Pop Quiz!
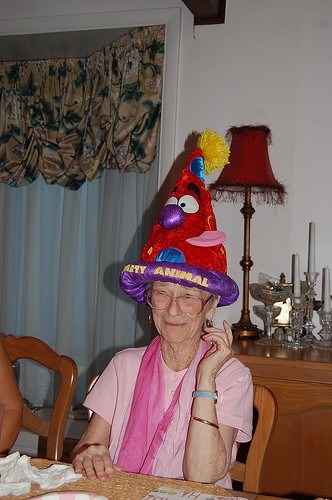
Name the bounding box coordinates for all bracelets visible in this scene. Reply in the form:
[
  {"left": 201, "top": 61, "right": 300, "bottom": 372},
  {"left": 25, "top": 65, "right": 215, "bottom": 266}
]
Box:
[{"left": 190, "top": 415, "right": 221, "bottom": 430}]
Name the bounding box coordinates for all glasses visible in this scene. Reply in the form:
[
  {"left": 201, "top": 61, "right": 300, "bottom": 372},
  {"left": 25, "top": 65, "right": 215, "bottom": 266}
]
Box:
[{"left": 147, "top": 283, "right": 213, "bottom": 316}]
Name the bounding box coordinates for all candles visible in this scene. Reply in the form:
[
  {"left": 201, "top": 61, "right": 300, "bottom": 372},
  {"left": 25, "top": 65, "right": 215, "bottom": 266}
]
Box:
[
  {"left": 309, "top": 220, "right": 315, "bottom": 274},
  {"left": 294, "top": 251, "right": 301, "bottom": 298},
  {"left": 322, "top": 266, "right": 331, "bottom": 312}
]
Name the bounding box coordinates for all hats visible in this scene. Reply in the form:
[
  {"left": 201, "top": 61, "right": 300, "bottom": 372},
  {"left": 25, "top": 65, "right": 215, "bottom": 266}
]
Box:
[{"left": 119, "top": 129, "right": 239, "bottom": 308}]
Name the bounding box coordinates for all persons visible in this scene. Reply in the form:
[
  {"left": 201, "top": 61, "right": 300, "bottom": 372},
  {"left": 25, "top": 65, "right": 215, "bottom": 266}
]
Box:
[
  {"left": 0, "top": 335, "right": 23, "bottom": 455},
  {"left": 68, "top": 265, "right": 255, "bottom": 490}
]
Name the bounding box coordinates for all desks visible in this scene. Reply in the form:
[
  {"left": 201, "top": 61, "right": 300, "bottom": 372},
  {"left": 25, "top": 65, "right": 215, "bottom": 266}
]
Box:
[
  {"left": 231, "top": 340, "right": 332, "bottom": 498},
  {"left": 0, "top": 454, "right": 275, "bottom": 500}
]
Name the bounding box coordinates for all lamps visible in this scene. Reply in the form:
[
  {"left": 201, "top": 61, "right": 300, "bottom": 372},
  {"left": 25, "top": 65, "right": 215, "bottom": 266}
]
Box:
[{"left": 207, "top": 122, "right": 288, "bottom": 339}]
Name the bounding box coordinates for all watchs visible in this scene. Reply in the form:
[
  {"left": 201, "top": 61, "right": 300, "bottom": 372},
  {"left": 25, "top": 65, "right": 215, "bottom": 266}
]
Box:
[{"left": 190, "top": 389, "right": 218, "bottom": 404}]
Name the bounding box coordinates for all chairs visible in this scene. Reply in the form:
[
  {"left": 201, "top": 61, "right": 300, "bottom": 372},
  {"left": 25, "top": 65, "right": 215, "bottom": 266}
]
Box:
[
  {"left": 3, "top": 334, "right": 79, "bottom": 460},
  {"left": 231, "top": 384, "right": 278, "bottom": 494}
]
